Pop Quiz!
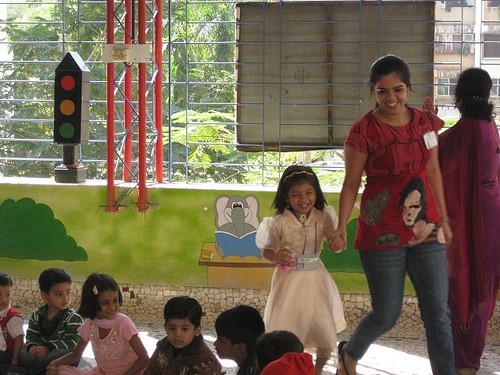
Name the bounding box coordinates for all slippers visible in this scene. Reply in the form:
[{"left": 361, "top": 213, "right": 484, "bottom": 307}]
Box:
[{"left": 335, "top": 340, "right": 356, "bottom": 375}]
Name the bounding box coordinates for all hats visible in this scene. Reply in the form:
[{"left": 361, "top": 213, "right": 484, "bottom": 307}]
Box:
[{"left": 260, "top": 353, "right": 315, "bottom": 375}]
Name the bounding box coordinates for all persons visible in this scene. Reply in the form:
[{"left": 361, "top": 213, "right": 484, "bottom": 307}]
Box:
[
  {"left": 328, "top": 53, "right": 457, "bottom": 375},
  {"left": 255, "top": 165, "right": 347, "bottom": 375},
  {"left": 213, "top": 305, "right": 265, "bottom": 375},
  {"left": 0, "top": 272, "right": 25, "bottom": 375},
  {"left": 423, "top": 68, "right": 500, "bottom": 375},
  {"left": 143, "top": 296, "right": 226, "bottom": 375},
  {"left": 18, "top": 268, "right": 85, "bottom": 375},
  {"left": 254, "top": 330, "right": 315, "bottom": 375},
  {"left": 46, "top": 273, "right": 149, "bottom": 375}
]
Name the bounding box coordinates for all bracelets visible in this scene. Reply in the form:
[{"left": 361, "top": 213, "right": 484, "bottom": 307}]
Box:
[{"left": 440, "top": 216, "right": 450, "bottom": 223}]
